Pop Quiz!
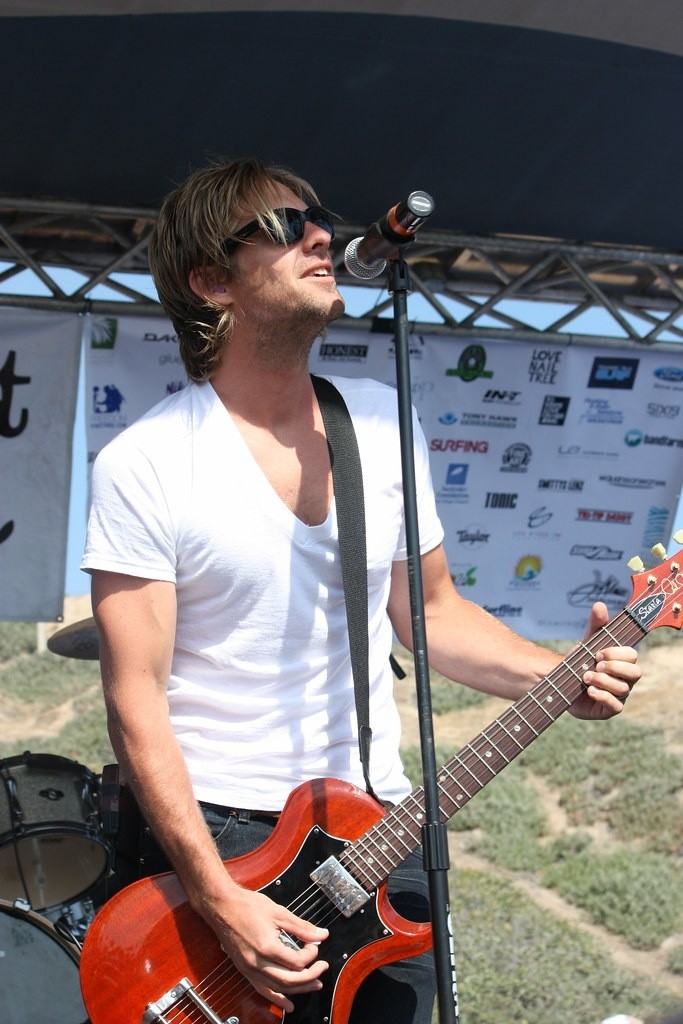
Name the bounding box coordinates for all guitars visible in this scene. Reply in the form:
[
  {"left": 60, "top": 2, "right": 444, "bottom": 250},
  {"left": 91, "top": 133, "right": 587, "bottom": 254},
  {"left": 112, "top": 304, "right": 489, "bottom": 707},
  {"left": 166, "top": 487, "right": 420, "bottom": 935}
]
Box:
[{"left": 79, "top": 527, "right": 683, "bottom": 1024}]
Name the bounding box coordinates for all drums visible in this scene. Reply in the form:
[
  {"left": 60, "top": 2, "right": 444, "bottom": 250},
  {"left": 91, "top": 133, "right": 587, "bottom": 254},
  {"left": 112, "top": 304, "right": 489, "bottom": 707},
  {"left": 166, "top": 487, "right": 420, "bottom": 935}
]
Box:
[
  {"left": 0, "top": 897, "right": 92, "bottom": 1024},
  {"left": 0, "top": 750, "right": 113, "bottom": 914}
]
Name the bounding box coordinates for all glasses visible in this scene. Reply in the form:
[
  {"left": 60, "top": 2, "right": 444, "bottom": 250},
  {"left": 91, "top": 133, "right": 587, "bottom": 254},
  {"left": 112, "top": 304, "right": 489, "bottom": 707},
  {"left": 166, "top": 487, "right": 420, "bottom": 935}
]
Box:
[{"left": 220, "top": 206, "right": 336, "bottom": 254}]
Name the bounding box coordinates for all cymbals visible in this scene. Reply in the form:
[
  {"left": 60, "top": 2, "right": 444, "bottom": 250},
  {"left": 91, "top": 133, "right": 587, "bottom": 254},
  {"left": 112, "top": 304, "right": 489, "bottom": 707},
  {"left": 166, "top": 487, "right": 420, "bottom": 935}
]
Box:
[{"left": 47, "top": 615, "right": 100, "bottom": 661}]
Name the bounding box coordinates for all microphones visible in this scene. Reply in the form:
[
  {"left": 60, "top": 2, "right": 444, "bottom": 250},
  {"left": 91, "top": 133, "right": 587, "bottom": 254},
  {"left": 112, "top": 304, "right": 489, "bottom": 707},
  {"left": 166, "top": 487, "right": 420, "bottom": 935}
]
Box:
[{"left": 344, "top": 190, "right": 435, "bottom": 279}]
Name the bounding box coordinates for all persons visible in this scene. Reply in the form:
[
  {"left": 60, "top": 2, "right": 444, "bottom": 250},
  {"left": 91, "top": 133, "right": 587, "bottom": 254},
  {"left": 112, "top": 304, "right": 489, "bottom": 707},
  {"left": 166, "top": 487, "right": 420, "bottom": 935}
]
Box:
[{"left": 80, "top": 160, "right": 642, "bottom": 1024}]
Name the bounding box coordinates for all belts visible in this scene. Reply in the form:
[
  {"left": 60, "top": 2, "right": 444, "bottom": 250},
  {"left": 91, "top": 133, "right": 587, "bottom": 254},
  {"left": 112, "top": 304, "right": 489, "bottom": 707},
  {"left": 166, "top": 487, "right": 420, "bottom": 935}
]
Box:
[{"left": 200, "top": 800, "right": 282, "bottom": 818}]
